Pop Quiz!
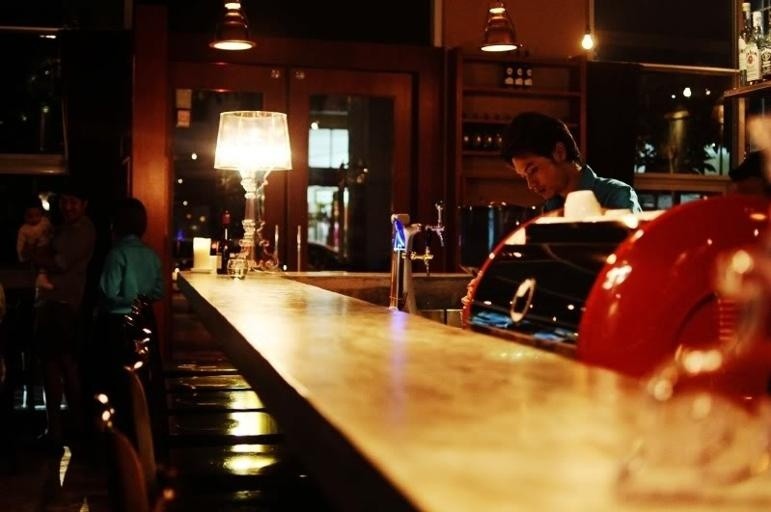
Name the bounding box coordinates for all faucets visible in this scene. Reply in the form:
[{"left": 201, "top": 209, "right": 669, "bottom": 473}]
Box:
[{"left": 390, "top": 197, "right": 448, "bottom": 312}]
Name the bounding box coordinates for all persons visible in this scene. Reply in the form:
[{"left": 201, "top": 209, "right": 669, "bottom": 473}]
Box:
[
  {"left": 501, "top": 111, "right": 643, "bottom": 213},
  {"left": 33, "top": 188, "right": 101, "bottom": 447},
  {"left": 14, "top": 201, "right": 58, "bottom": 291},
  {"left": 100, "top": 197, "right": 170, "bottom": 423}
]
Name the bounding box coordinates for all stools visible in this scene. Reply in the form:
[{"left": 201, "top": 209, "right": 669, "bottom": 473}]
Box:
[{"left": 168, "top": 347, "right": 316, "bottom": 511}]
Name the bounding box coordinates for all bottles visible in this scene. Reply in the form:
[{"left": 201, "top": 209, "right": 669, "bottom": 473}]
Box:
[
  {"left": 217, "top": 214, "right": 235, "bottom": 274},
  {"left": 738, "top": 2, "right": 754, "bottom": 88},
  {"left": 745, "top": 11, "right": 771, "bottom": 85}
]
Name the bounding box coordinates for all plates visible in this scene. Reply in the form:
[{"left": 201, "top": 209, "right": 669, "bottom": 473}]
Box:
[{"left": 191, "top": 268, "right": 213, "bottom": 273}]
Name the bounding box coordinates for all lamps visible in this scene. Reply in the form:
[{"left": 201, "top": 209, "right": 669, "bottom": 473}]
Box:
[
  {"left": 213, "top": 105, "right": 296, "bottom": 271},
  {"left": 477, "top": 0, "right": 522, "bottom": 56},
  {"left": 206, "top": 0, "right": 257, "bottom": 54},
  {"left": 579, "top": 0, "right": 596, "bottom": 52}
]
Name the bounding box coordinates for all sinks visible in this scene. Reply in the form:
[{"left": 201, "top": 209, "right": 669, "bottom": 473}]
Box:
[{"left": 278, "top": 267, "right": 477, "bottom": 315}]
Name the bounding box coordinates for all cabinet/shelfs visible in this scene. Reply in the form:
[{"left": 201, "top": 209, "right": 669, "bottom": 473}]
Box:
[
  {"left": 723, "top": 0, "right": 771, "bottom": 185},
  {"left": 451, "top": 51, "right": 591, "bottom": 272},
  {"left": 585, "top": 59, "right": 745, "bottom": 207}
]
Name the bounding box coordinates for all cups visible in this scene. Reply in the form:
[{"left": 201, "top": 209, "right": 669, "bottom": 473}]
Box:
[
  {"left": 192, "top": 237, "right": 211, "bottom": 268},
  {"left": 227, "top": 259, "right": 248, "bottom": 279}
]
